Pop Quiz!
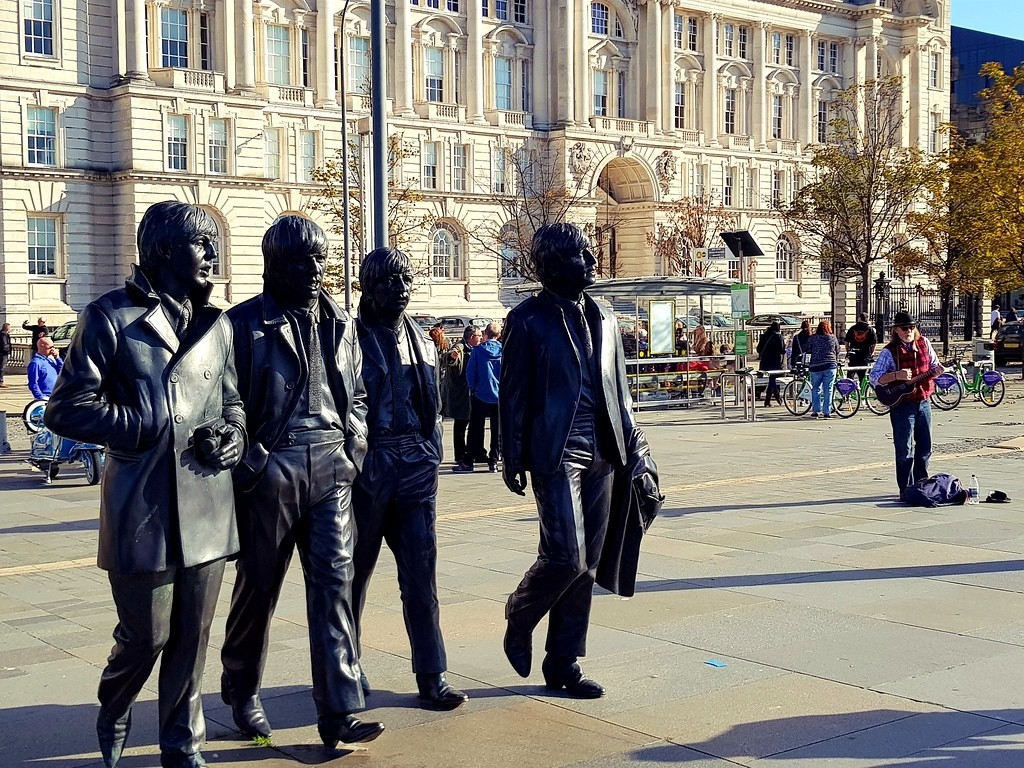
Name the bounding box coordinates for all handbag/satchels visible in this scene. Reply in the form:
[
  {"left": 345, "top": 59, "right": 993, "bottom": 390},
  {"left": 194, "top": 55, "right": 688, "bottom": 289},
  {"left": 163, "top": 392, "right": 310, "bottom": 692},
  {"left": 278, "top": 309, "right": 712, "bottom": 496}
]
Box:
[{"left": 903, "top": 473, "right": 970, "bottom": 507}]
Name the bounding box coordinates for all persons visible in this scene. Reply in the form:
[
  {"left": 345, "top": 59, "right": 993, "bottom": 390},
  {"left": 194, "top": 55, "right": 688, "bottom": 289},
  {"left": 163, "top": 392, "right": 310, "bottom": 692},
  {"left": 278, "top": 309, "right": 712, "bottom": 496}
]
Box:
[
  {"left": 756, "top": 321, "right": 786, "bottom": 408},
  {"left": 225, "top": 216, "right": 385, "bottom": 750},
  {"left": 22, "top": 316, "right": 49, "bottom": 361},
  {"left": 27, "top": 337, "right": 64, "bottom": 457},
  {"left": 42, "top": 200, "right": 249, "bottom": 768},
  {"left": 622, "top": 322, "right": 743, "bottom": 397},
  {"left": 350, "top": 247, "right": 469, "bottom": 705},
  {"left": 805, "top": 321, "right": 841, "bottom": 420},
  {"left": 844, "top": 313, "right": 877, "bottom": 387},
  {"left": 868, "top": 312, "right": 945, "bottom": 501},
  {"left": 430, "top": 322, "right": 502, "bottom": 473},
  {"left": 499, "top": 223, "right": 665, "bottom": 697},
  {"left": 990, "top": 304, "right": 1018, "bottom": 339},
  {"left": 0, "top": 323, "right": 12, "bottom": 388},
  {"left": 790, "top": 320, "right": 812, "bottom": 407}
]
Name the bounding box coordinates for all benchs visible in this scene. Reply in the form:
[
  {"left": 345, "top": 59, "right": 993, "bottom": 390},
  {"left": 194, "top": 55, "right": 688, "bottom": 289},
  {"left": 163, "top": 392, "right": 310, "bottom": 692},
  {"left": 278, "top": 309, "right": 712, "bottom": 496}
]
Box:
[{"left": 629, "top": 369, "right": 728, "bottom": 408}]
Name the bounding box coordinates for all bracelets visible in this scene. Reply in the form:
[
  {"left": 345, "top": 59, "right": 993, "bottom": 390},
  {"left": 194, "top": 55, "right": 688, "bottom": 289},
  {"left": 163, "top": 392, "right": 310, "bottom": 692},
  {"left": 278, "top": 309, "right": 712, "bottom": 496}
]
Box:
[{"left": 894, "top": 372, "right": 897, "bottom": 380}]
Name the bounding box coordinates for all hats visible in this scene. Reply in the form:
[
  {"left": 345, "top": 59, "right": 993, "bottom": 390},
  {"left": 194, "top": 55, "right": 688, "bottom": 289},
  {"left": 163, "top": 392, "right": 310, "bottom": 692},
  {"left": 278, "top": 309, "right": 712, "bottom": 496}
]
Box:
[{"left": 892, "top": 312, "right": 919, "bottom": 325}]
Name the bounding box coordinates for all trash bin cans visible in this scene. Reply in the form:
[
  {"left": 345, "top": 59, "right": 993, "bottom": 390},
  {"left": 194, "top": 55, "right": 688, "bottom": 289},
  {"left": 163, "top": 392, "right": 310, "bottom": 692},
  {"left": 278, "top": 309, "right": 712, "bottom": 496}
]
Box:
[
  {"left": 972, "top": 337, "right": 995, "bottom": 383},
  {"left": 0, "top": 411, "right": 11, "bottom": 454}
]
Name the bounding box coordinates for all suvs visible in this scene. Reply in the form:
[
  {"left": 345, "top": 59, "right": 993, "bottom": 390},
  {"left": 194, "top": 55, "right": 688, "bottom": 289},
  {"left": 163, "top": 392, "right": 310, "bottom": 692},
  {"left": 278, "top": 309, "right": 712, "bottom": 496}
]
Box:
[{"left": 411, "top": 314, "right": 498, "bottom": 346}]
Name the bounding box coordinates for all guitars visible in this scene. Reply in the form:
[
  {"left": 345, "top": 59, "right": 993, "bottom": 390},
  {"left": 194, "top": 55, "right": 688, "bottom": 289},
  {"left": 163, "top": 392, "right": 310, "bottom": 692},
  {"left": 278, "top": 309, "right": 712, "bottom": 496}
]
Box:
[{"left": 875, "top": 358, "right": 961, "bottom": 408}]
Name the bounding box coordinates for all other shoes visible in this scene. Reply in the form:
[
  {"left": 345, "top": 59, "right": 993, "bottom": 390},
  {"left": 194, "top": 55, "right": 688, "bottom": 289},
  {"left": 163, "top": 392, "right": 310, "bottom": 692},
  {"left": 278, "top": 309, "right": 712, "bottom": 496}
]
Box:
[
  {"left": 0, "top": 384, "right": 8, "bottom": 389},
  {"left": 823, "top": 414, "right": 834, "bottom": 419},
  {"left": 475, "top": 456, "right": 490, "bottom": 462},
  {"left": 811, "top": 413, "right": 818, "bottom": 419},
  {"left": 457, "top": 459, "right": 463, "bottom": 464}
]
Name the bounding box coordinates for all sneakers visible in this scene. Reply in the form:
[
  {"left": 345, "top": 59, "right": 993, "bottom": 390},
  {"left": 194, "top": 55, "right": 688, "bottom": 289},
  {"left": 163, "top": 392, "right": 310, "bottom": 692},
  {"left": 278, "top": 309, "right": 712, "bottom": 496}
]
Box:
[
  {"left": 451, "top": 463, "right": 474, "bottom": 472},
  {"left": 490, "top": 467, "right": 498, "bottom": 473}
]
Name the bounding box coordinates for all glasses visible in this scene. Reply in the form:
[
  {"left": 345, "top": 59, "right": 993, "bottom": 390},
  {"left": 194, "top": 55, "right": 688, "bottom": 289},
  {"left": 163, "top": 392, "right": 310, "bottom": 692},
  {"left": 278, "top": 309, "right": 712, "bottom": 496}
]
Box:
[
  {"left": 474, "top": 334, "right": 481, "bottom": 336},
  {"left": 897, "top": 325, "right": 915, "bottom": 331}
]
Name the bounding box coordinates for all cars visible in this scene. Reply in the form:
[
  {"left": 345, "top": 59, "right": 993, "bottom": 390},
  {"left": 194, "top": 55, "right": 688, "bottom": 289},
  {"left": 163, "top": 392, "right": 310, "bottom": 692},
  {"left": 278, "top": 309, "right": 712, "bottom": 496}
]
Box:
[
  {"left": 993, "top": 310, "right": 1024, "bottom": 367},
  {"left": 50, "top": 321, "right": 78, "bottom": 353},
  {"left": 675, "top": 314, "right": 734, "bottom": 330},
  {"left": 745, "top": 314, "right": 803, "bottom": 326}
]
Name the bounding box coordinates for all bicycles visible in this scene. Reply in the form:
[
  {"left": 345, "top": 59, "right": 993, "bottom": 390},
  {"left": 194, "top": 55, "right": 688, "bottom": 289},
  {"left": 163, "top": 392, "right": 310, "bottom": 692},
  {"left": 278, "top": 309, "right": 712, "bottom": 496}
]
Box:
[
  {"left": 783, "top": 361, "right": 861, "bottom": 418},
  {"left": 861, "top": 344, "right": 1006, "bottom": 416}
]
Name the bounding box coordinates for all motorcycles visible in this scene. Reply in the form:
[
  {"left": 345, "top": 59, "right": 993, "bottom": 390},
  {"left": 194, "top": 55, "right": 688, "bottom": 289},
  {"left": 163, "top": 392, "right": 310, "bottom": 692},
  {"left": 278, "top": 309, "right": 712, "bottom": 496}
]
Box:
[{"left": 23, "top": 398, "right": 104, "bottom": 484}]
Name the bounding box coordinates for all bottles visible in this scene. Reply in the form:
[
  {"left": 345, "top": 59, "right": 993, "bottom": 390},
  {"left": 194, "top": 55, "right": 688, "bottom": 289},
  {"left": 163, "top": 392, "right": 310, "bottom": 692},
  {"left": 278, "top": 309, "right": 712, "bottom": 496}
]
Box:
[{"left": 968, "top": 474, "right": 980, "bottom": 505}]
